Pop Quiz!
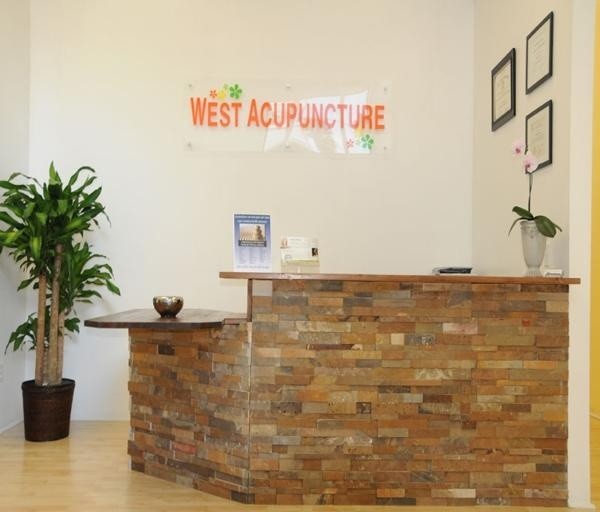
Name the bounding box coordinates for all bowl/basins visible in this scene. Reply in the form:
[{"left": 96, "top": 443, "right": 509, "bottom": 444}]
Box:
[{"left": 153, "top": 296, "right": 183, "bottom": 318}]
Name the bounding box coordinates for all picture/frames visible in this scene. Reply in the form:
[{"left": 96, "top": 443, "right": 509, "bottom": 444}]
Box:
[{"left": 489, "top": 10, "right": 555, "bottom": 175}]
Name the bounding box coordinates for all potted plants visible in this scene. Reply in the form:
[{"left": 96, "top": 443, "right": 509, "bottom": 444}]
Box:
[{"left": 0, "top": 159, "right": 122, "bottom": 443}]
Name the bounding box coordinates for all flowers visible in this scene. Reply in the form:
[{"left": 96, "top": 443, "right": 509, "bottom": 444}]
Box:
[{"left": 506, "top": 141, "right": 562, "bottom": 239}]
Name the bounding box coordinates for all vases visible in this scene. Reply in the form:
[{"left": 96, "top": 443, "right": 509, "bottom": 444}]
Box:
[{"left": 520, "top": 222, "right": 547, "bottom": 276}]
